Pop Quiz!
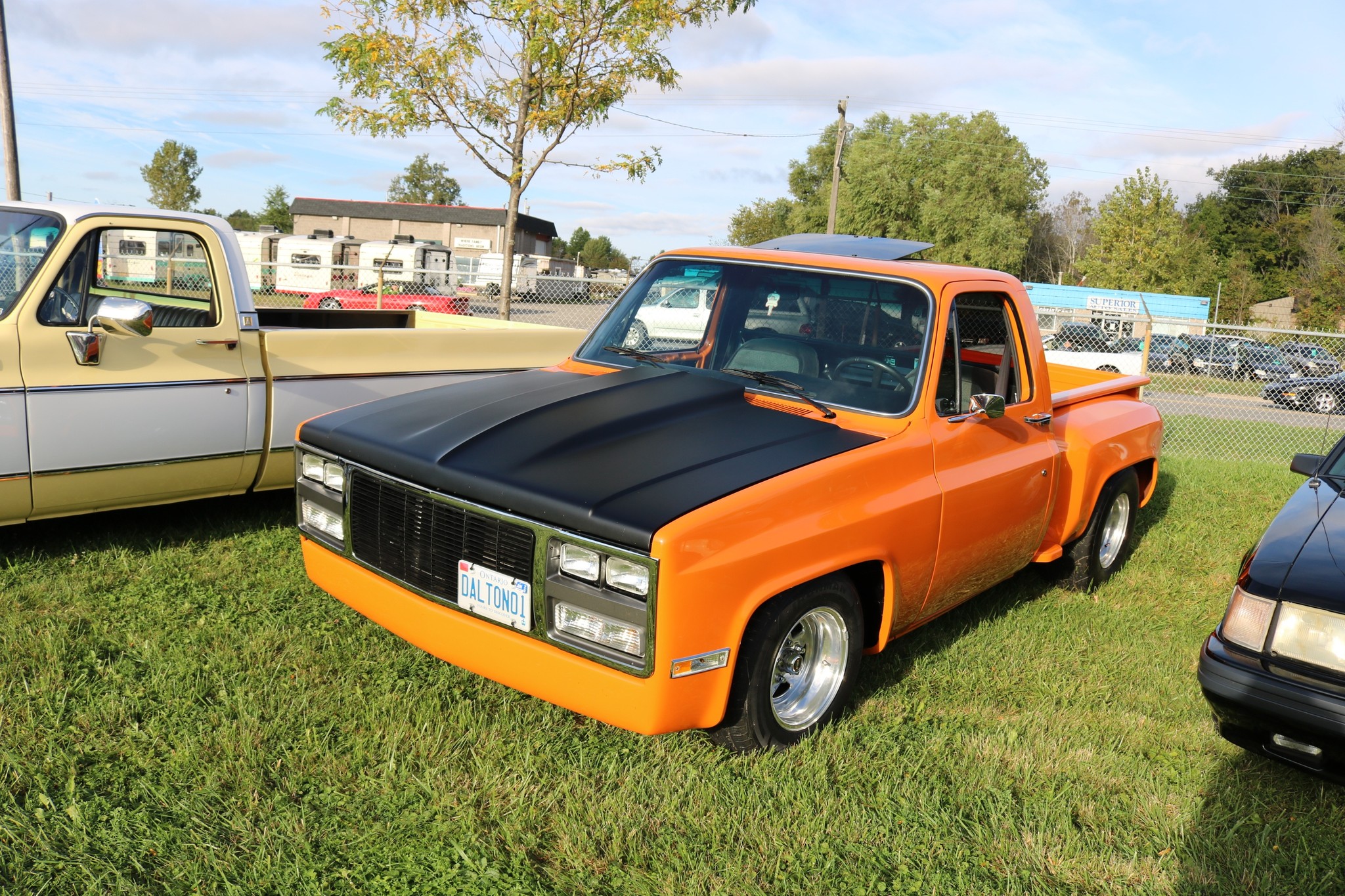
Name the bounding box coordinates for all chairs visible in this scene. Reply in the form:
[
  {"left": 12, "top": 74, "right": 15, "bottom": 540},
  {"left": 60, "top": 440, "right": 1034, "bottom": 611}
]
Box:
[
  {"left": 722, "top": 337, "right": 819, "bottom": 384},
  {"left": 894, "top": 364, "right": 999, "bottom": 412}
]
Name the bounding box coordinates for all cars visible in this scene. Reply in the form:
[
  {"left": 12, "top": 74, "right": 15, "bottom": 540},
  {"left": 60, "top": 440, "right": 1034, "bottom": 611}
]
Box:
[
  {"left": 967, "top": 320, "right": 1319, "bottom": 383},
  {"left": 1262, "top": 371, "right": 1345, "bottom": 414},
  {"left": 303, "top": 281, "right": 473, "bottom": 316},
  {"left": 1197, "top": 435, "right": 1345, "bottom": 771}
]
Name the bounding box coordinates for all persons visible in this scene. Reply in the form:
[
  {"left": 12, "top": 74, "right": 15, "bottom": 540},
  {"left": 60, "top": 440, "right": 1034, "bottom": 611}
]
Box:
[
  {"left": 1057, "top": 341, "right": 1071, "bottom": 351},
  {"left": 382, "top": 284, "right": 400, "bottom": 295}
]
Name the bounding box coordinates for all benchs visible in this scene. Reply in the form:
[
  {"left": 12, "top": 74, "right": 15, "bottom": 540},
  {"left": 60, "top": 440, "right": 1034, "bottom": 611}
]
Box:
[{"left": 62, "top": 292, "right": 209, "bottom": 328}]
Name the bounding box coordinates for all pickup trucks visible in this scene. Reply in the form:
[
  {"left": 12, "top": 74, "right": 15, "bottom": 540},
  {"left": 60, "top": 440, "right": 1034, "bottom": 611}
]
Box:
[
  {"left": 0, "top": 202, "right": 590, "bottom": 528},
  {"left": 292, "top": 247, "right": 1165, "bottom": 756}
]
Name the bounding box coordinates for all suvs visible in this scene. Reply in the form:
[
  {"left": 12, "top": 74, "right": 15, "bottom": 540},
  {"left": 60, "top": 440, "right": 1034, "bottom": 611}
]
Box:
[
  {"left": 620, "top": 283, "right": 816, "bottom": 352},
  {"left": 1280, "top": 340, "right": 1343, "bottom": 378}
]
